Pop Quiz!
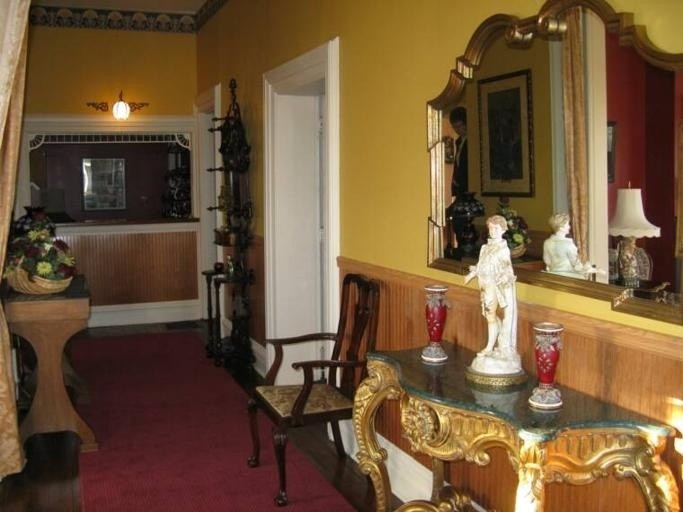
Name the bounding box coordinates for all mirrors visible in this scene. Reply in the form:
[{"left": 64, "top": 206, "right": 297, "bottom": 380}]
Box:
[{"left": 425, "top": 0, "right": 683, "bottom": 328}]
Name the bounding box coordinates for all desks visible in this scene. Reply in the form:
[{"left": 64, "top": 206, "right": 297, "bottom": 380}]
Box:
[
  {"left": 352, "top": 338, "right": 683, "bottom": 512},
  {"left": 611, "top": 278, "right": 675, "bottom": 305},
  {"left": 3, "top": 274, "right": 99, "bottom": 454},
  {"left": 463, "top": 250, "right": 545, "bottom": 272},
  {"left": 202, "top": 270, "right": 254, "bottom": 368}
]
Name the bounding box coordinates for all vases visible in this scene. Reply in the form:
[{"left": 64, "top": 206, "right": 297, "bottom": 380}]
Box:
[
  {"left": 15, "top": 206, "right": 55, "bottom": 237},
  {"left": 447, "top": 191, "right": 484, "bottom": 258}
]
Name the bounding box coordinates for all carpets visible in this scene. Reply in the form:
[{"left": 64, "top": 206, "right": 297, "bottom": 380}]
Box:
[{"left": 72, "top": 332, "right": 357, "bottom": 512}]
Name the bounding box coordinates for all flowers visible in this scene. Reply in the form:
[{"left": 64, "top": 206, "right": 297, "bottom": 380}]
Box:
[
  {"left": 484, "top": 197, "right": 532, "bottom": 250},
  {"left": 2, "top": 227, "right": 76, "bottom": 282}
]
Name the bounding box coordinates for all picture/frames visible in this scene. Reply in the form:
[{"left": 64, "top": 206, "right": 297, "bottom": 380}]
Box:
[
  {"left": 80, "top": 155, "right": 128, "bottom": 211},
  {"left": 477, "top": 68, "right": 535, "bottom": 197},
  {"left": 606, "top": 120, "right": 616, "bottom": 182}
]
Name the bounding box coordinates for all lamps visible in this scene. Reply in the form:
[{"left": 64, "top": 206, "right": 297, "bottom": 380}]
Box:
[
  {"left": 86, "top": 89, "right": 151, "bottom": 120},
  {"left": 610, "top": 181, "right": 660, "bottom": 288}
]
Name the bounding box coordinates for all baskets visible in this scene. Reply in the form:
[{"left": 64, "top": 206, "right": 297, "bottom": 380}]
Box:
[{"left": 4, "top": 244, "right": 75, "bottom": 296}]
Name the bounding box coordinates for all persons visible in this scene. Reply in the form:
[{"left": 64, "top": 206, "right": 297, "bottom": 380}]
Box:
[
  {"left": 460, "top": 214, "right": 518, "bottom": 361},
  {"left": 541, "top": 213, "right": 597, "bottom": 281},
  {"left": 446, "top": 105, "right": 468, "bottom": 206},
  {"left": 494, "top": 195, "right": 516, "bottom": 222}
]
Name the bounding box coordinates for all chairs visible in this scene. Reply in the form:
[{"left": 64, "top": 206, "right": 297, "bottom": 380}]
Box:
[{"left": 247, "top": 273, "right": 380, "bottom": 507}]
